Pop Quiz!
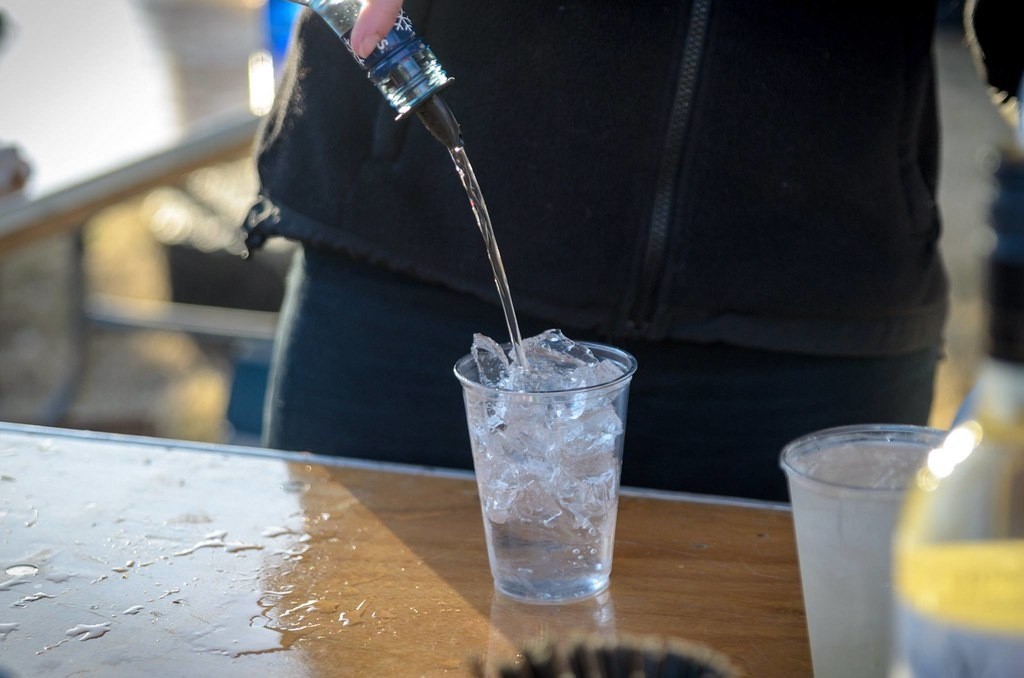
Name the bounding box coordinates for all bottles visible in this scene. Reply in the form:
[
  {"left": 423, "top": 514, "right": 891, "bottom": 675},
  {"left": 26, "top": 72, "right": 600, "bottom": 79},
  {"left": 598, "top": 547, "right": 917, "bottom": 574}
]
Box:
[
  {"left": 888, "top": 148, "right": 1023, "bottom": 677},
  {"left": 290, "top": 0, "right": 463, "bottom": 155}
]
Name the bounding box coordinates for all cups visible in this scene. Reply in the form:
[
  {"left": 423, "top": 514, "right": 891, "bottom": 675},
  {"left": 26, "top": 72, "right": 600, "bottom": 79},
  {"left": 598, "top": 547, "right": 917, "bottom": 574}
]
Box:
[
  {"left": 780, "top": 422, "right": 945, "bottom": 677},
  {"left": 453, "top": 343, "right": 637, "bottom": 606}
]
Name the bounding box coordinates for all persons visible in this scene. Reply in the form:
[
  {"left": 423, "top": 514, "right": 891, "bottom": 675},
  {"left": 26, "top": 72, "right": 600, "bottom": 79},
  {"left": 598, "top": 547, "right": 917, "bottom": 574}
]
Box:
[{"left": 236, "top": 0, "right": 960, "bottom": 515}]
